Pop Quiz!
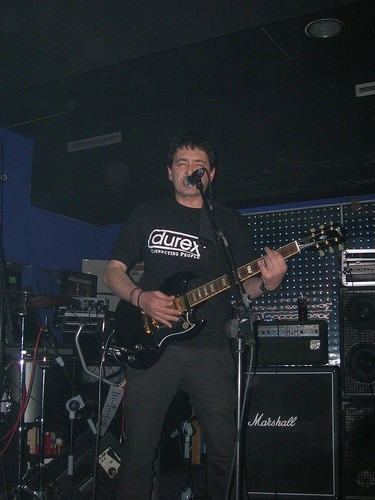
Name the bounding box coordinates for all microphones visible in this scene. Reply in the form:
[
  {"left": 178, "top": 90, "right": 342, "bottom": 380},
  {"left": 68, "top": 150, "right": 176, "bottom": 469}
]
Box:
[{"left": 184, "top": 168, "right": 206, "bottom": 188}]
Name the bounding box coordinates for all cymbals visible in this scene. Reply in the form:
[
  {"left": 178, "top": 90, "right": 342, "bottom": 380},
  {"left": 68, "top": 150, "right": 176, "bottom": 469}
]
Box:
[{"left": 1, "top": 289, "right": 82, "bottom": 309}]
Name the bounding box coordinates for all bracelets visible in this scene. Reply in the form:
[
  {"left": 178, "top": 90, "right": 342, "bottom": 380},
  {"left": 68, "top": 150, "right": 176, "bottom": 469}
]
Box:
[
  {"left": 260, "top": 282, "right": 281, "bottom": 294},
  {"left": 129, "top": 287, "right": 145, "bottom": 309}
]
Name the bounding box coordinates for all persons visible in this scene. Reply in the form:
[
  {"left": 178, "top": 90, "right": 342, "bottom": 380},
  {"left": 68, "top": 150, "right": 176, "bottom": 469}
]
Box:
[{"left": 102, "top": 133, "right": 287, "bottom": 500}]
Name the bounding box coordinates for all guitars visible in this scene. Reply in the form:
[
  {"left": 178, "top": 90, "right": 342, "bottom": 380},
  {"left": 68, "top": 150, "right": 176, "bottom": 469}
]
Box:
[{"left": 111, "top": 219, "right": 346, "bottom": 367}]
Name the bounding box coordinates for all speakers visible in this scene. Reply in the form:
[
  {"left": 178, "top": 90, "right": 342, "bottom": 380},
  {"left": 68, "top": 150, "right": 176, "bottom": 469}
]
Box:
[
  {"left": 236, "top": 287, "right": 375, "bottom": 500},
  {"left": 20, "top": 424, "right": 123, "bottom": 500}
]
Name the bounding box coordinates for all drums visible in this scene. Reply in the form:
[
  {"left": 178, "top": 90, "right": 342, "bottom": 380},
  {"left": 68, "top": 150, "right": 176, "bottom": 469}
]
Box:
[{"left": 0, "top": 343, "right": 67, "bottom": 425}]
